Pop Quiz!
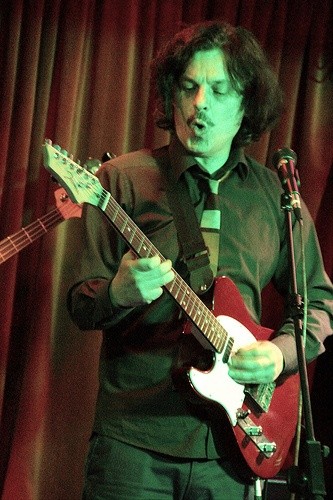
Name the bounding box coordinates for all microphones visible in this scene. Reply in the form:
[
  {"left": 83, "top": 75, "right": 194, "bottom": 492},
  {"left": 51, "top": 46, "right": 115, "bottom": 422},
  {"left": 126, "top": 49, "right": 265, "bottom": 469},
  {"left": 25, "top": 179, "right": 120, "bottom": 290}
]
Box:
[{"left": 271, "top": 148, "right": 303, "bottom": 220}]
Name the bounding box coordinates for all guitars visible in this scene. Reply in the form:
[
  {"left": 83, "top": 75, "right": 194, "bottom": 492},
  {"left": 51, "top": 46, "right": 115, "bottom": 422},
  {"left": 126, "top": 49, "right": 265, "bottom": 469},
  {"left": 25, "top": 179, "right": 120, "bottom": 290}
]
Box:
[
  {"left": 41, "top": 138, "right": 316, "bottom": 478},
  {"left": 0, "top": 150, "right": 113, "bottom": 265}
]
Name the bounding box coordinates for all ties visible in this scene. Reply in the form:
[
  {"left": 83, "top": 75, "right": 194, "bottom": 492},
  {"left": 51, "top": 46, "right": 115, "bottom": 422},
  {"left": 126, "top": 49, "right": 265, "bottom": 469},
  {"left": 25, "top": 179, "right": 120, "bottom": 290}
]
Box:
[{"left": 188, "top": 163, "right": 239, "bottom": 314}]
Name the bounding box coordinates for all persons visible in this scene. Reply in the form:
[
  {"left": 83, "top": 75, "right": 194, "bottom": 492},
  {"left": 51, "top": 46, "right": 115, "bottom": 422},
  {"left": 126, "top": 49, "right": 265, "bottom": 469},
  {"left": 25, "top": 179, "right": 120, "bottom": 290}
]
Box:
[{"left": 67, "top": 21, "right": 332, "bottom": 499}]
